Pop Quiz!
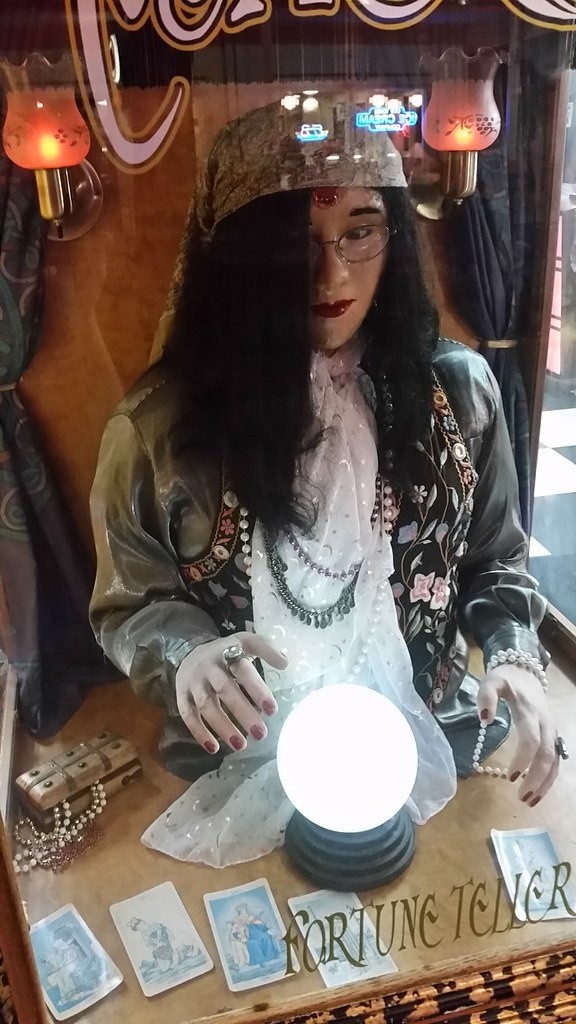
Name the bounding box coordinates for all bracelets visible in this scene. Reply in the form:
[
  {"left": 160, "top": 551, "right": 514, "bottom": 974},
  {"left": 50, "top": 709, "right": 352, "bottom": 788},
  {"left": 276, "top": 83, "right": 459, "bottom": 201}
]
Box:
[{"left": 471, "top": 647, "right": 548, "bottom": 778}]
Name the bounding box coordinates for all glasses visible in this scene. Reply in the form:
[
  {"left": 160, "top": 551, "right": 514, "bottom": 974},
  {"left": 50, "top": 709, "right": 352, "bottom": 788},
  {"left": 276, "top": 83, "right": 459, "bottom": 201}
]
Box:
[{"left": 309, "top": 224, "right": 396, "bottom": 272}]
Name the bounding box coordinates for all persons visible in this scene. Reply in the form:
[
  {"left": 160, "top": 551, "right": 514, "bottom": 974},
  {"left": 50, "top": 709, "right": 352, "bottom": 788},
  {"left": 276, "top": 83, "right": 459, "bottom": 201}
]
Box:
[{"left": 89, "top": 96, "right": 561, "bottom": 808}]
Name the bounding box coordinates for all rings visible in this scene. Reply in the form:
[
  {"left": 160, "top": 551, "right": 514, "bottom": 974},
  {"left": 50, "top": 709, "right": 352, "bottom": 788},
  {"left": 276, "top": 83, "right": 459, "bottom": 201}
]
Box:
[
  {"left": 223, "top": 645, "right": 250, "bottom": 679},
  {"left": 555, "top": 737, "right": 569, "bottom": 760}
]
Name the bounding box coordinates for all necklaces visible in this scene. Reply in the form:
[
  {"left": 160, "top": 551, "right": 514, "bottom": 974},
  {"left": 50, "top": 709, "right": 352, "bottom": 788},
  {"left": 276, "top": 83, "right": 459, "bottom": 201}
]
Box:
[
  {"left": 237, "top": 369, "right": 396, "bottom": 705},
  {"left": 11, "top": 781, "right": 107, "bottom": 872}
]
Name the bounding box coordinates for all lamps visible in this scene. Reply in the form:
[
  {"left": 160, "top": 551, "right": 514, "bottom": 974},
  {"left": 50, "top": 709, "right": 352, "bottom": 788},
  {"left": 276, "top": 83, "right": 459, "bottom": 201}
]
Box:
[
  {"left": 0, "top": 47, "right": 106, "bottom": 241},
  {"left": 404, "top": 43, "right": 508, "bottom": 221},
  {"left": 276, "top": 679, "right": 418, "bottom": 893}
]
